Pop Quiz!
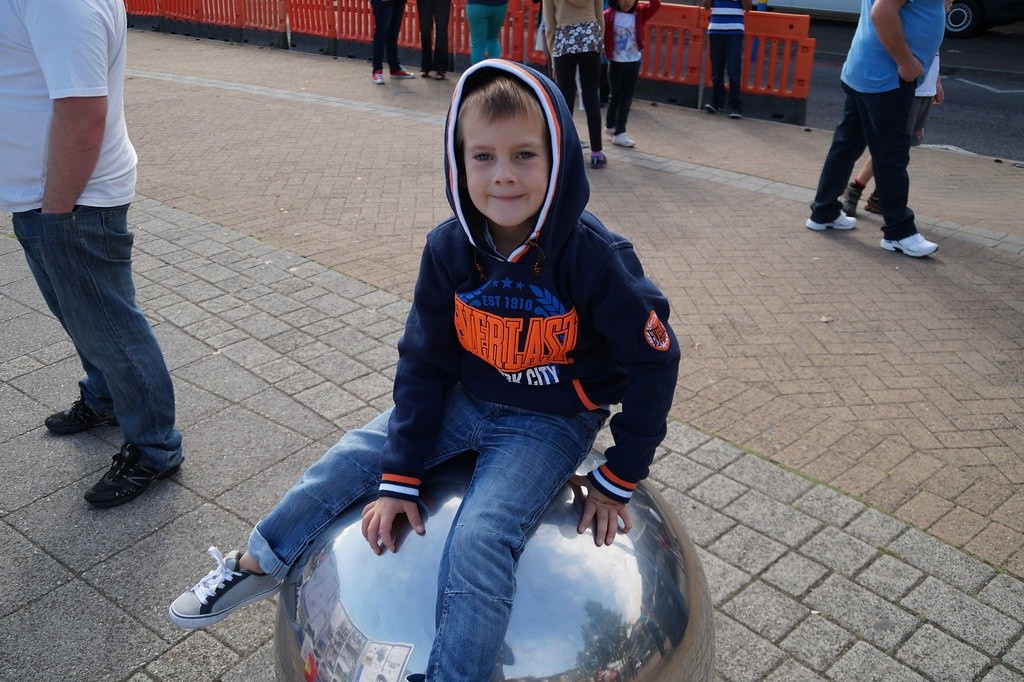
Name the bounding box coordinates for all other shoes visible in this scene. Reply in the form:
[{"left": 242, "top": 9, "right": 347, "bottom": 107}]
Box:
[
  {"left": 730, "top": 108, "right": 743, "bottom": 117},
  {"left": 421, "top": 70, "right": 445, "bottom": 79},
  {"left": 705, "top": 101, "right": 725, "bottom": 112}
]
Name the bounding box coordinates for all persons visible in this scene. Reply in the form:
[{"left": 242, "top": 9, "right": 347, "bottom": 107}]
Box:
[
  {"left": 603, "top": 0, "right": 661, "bottom": 148},
  {"left": 806, "top": 0, "right": 953, "bottom": 257},
  {"left": 841, "top": 47, "right": 944, "bottom": 216},
  {"left": 532, "top": 0, "right": 610, "bottom": 110},
  {"left": 705, "top": 0, "right": 753, "bottom": 118},
  {"left": 417, "top": 0, "right": 451, "bottom": 79},
  {"left": 466, "top": 0, "right": 508, "bottom": 66},
  {"left": 543, "top": 0, "right": 607, "bottom": 169},
  {"left": 0, "top": 0, "right": 185, "bottom": 509},
  {"left": 168, "top": 59, "right": 680, "bottom": 682},
  {"left": 370, "top": 0, "right": 415, "bottom": 84}
]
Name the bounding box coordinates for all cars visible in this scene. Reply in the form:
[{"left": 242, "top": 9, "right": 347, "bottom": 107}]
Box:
[{"left": 747, "top": 0, "right": 1024, "bottom": 41}]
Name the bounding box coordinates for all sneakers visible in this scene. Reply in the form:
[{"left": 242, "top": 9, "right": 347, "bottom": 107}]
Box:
[
  {"left": 612, "top": 133, "right": 636, "bottom": 147},
  {"left": 372, "top": 70, "right": 384, "bottom": 84},
  {"left": 389, "top": 68, "right": 416, "bottom": 78},
  {"left": 169, "top": 547, "right": 283, "bottom": 628},
  {"left": 84, "top": 443, "right": 181, "bottom": 509},
  {"left": 841, "top": 185, "right": 861, "bottom": 218},
  {"left": 864, "top": 194, "right": 883, "bottom": 214},
  {"left": 44, "top": 396, "right": 119, "bottom": 435},
  {"left": 591, "top": 152, "right": 607, "bottom": 169},
  {"left": 880, "top": 233, "right": 939, "bottom": 257},
  {"left": 604, "top": 127, "right": 615, "bottom": 136},
  {"left": 806, "top": 209, "right": 857, "bottom": 231}
]
